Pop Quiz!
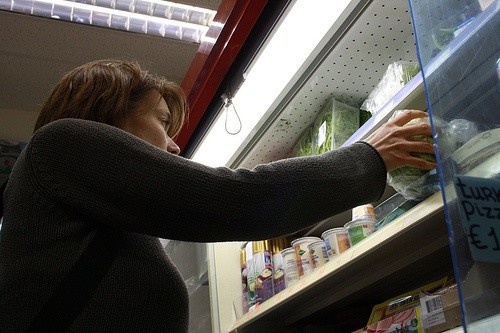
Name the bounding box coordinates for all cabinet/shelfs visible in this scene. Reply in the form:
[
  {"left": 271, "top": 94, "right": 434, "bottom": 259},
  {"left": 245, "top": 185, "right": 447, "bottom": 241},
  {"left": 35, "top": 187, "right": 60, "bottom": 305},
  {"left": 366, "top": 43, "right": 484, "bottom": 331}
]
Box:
[{"left": 212, "top": 0, "right": 500, "bottom": 333}]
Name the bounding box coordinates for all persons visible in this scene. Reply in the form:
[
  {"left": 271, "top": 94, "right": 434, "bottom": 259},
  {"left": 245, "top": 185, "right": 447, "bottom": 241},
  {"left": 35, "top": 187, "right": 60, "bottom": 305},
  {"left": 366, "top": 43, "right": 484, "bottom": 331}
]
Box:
[{"left": 0, "top": 60, "right": 442, "bottom": 333}]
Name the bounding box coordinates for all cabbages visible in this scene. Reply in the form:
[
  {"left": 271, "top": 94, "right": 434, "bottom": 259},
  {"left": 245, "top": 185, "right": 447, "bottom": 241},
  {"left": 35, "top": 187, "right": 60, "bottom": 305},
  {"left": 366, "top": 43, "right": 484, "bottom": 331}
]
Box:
[{"left": 382, "top": 109, "right": 455, "bottom": 198}]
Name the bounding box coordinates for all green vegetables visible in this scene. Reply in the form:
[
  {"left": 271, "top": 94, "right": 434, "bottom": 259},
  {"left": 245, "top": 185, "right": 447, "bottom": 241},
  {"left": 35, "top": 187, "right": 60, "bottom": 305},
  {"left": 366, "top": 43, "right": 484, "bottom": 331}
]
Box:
[{"left": 287, "top": 97, "right": 374, "bottom": 157}]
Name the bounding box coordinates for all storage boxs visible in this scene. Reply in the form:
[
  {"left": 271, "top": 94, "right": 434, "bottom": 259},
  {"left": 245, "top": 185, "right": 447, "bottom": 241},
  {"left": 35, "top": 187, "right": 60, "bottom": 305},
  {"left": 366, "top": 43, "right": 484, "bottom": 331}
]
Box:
[{"left": 422, "top": 260, "right": 500, "bottom": 333}]
